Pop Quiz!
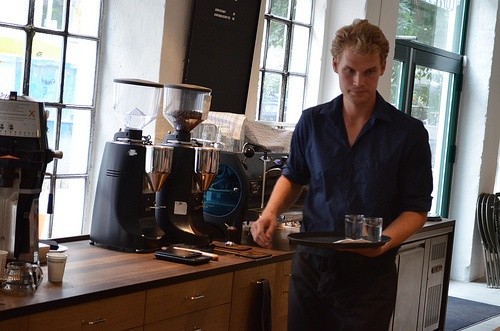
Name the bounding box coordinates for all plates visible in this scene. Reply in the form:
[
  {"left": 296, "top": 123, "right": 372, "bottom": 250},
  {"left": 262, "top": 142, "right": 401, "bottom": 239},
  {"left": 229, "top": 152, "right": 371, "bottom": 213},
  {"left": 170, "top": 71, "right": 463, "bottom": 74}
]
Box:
[{"left": 49, "top": 245, "right": 69, "bottom": 252}]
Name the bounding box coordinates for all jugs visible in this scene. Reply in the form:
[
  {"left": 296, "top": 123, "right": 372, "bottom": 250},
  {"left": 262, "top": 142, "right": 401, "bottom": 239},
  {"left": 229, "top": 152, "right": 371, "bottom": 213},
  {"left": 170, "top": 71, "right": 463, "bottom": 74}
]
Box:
[{"left": 1, "top": 262, "right": 44, "bottom": 296}]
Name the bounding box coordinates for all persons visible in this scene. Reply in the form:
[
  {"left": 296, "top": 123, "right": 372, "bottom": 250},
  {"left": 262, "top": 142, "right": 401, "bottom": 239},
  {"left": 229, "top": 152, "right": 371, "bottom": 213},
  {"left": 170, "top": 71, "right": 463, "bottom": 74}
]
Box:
[{"left": 250, "top": 17, "right": 433, "bottom": 331}]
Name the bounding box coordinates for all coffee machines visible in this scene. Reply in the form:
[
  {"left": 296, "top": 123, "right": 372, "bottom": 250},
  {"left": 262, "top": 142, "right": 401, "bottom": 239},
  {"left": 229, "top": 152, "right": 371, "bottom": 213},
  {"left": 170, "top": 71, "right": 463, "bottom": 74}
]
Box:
[
  {"left": 156, "top": 83, "right": 222, "bottom": 248},
  {"left": 0, "top": 91, "right": 64, "bottom": 267},
  {"left": 89, "top": 77, "right": 175, "bottom": 253}
]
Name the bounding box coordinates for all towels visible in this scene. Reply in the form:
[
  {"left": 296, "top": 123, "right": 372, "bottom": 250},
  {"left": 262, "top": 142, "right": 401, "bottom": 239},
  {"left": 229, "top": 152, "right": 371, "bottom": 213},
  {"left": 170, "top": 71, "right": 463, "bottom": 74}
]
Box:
[{"left": 256, "top": 279, "right": 271, "bottom": 331}]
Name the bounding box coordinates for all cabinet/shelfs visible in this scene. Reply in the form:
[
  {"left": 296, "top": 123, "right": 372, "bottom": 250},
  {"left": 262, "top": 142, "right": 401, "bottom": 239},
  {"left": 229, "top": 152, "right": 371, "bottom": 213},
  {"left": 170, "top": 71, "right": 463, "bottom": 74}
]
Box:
[{"left": 0, "top": 260, "right": 292, "bottom": 331}]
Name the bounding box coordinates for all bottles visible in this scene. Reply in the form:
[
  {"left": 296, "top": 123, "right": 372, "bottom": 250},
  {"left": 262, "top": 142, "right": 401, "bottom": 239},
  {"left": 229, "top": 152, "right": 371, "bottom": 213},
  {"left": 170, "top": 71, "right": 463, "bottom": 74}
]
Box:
[{"left": 30, "top": 251, "right": 41, "bottom": 289}]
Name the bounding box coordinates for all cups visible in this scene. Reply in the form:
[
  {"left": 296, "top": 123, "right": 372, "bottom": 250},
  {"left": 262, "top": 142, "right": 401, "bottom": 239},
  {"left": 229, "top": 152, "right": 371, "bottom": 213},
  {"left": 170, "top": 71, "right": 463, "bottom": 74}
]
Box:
[
  {"left": 46, "top": 252, "right": 68, "bottom": 282},
  {"left": 0, "top": 250, "right": 8, "bottom": 280},
  {"left": 345, "top": 214, "right": 364, "bottom": 241},
  {"left": 191, "top": 123, "right": 234, "bottom": 152},
  {"left": 363, "top": 217, "right": 383, "bottom": 242}
]
